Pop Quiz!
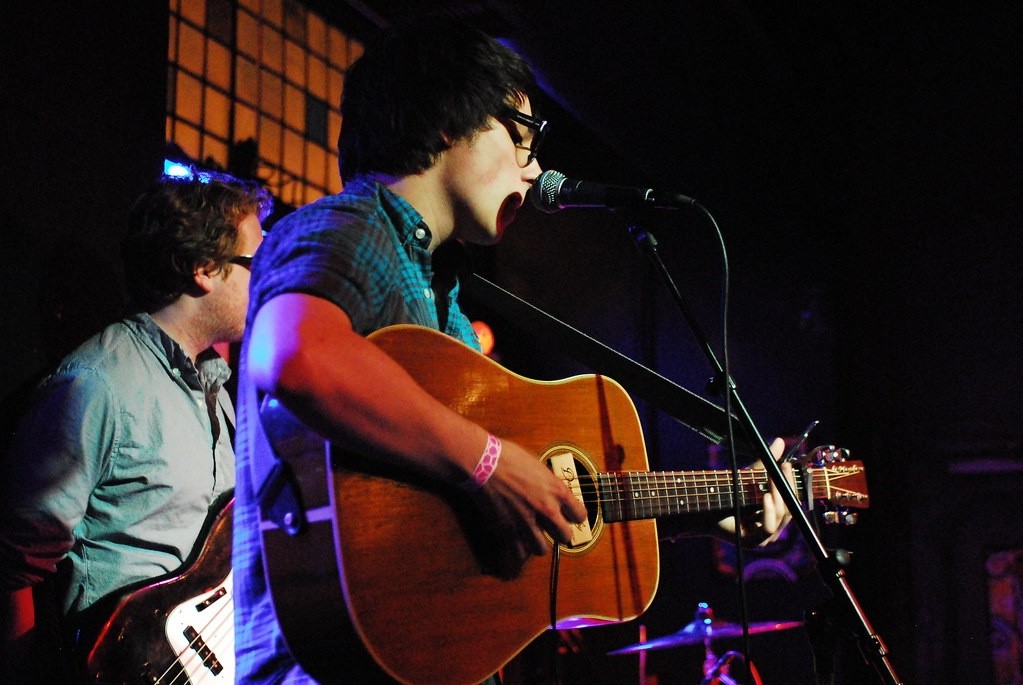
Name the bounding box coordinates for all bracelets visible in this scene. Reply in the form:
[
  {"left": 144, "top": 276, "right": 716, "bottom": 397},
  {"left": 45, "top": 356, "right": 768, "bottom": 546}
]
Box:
[{"left": 462, "top": 432, "right": 502, "bottom": 496}]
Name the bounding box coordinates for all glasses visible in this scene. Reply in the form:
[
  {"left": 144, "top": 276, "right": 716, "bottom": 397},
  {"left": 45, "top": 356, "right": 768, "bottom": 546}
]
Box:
[{"left": 492, "top": 101, "right": 548, "bottom": 156}]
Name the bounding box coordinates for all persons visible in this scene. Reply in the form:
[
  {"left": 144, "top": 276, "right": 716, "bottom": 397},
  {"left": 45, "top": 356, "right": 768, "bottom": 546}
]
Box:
[
  {"left": 231, "top": 31, "right": 801, "bottom": 685},
  {"left": 1, "top": 164, "right": 275, "bottom": 685}
]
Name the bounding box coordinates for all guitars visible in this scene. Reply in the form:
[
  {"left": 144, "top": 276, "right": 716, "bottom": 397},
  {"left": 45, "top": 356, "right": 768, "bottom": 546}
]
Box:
[
  {"left": 256, "top": 322, "right": 873, "bottom": 685},
  {"left": 61, "top": 486, "right": 235, "bottom": 685}
]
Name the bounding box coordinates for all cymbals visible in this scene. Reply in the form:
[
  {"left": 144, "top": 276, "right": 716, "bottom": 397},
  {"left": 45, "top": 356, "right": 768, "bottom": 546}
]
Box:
[
  {"left": 604, "top": 602, "right": 805, "bottom": 658},
  {"left": 547, "top": 615, "right": 626, "bottom": 630}
]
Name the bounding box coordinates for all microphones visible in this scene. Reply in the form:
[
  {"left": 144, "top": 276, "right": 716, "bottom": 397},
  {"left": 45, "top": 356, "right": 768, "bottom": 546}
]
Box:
[{"left": 530, "top": 170, "right": 697, "bottom": 214}]
